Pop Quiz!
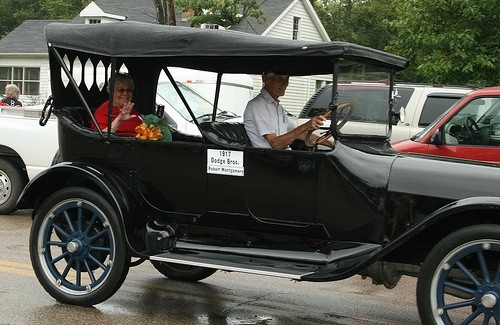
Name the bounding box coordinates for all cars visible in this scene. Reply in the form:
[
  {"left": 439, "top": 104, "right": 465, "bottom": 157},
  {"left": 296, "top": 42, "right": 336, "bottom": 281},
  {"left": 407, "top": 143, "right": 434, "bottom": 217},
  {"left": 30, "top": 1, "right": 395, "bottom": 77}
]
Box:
[{"left": 16, "top": 19, "right": 499, "bottom": 324}]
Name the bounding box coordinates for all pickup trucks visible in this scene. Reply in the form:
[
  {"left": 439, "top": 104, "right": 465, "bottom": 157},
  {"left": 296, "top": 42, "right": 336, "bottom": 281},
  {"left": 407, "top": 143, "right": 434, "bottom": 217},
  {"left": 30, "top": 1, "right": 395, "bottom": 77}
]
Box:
[{"left": 1, "top": 67, "right": 246, "bottom": 215}]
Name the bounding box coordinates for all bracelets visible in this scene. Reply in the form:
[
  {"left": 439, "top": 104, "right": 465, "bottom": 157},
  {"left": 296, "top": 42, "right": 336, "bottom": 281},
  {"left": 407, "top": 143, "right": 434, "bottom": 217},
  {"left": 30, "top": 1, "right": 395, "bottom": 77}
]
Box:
[{"left": 116, "top": 117, "right": 123, "bottom": 122}]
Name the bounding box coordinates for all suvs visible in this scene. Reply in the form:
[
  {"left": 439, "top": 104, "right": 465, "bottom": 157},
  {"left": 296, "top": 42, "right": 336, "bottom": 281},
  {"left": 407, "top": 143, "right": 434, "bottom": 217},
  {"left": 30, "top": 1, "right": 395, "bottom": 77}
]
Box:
[
  {"left": 390, "top": 87, "right": 500, "bottom": 163},
  {"left": 298, "top": 78, "right": 494, "bottom": 147}
]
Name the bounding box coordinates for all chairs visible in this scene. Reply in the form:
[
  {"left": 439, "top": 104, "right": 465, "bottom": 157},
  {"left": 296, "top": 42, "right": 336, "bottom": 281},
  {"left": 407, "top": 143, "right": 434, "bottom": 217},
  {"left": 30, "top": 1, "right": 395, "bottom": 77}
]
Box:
[{"left": 198, "top": 120, "right": 253, "bottom": 149}]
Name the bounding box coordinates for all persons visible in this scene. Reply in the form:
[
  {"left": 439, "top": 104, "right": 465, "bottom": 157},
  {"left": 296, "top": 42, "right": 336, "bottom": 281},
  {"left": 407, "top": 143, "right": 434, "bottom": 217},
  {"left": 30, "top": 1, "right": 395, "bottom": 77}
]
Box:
[
  {"left": 90, "top": 71, "right": 147, "bottom": 137},
  {"left": 244, "top": 64, "right": 334, "bottom": 151},
  {"left": 0, "top": 84, "right": 22, "bottom": 108}
]
